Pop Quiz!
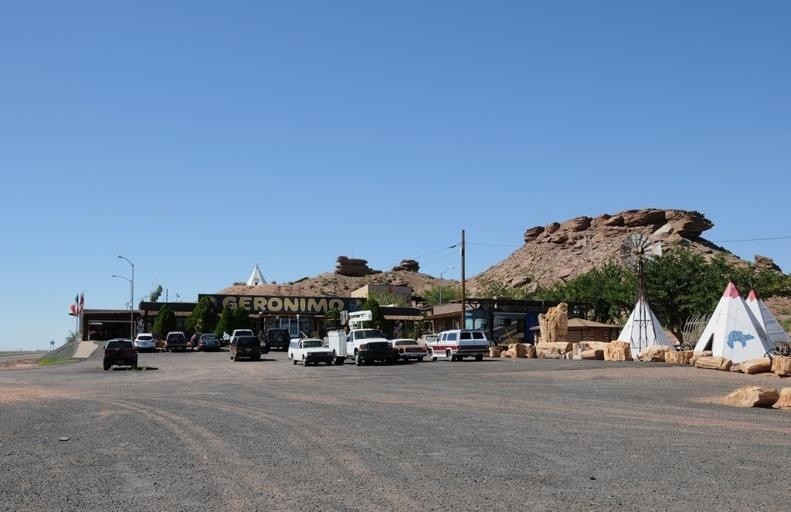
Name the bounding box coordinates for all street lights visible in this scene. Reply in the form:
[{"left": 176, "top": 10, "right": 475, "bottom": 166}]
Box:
[
  {"left": 117, "top": 253, "right": 137, "bottom": 345},
  {"left": 438, "top": 265, "right": 455, "bottom": 306},
  {"left": 109, "top": 272, "right": 135, "bottom": 311}
]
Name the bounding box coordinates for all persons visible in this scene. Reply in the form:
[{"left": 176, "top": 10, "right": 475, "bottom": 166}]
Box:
[
  {"left": 223, "top": 331, "right": 229, "bottom": 347},
  {"left": 190, "top": 333, "right": 196, "bottom": 350}
]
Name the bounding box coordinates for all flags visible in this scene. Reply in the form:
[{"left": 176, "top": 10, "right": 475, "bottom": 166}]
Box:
[{"left": 75, "top": 295, "right": 85, "bottom": 304}]
